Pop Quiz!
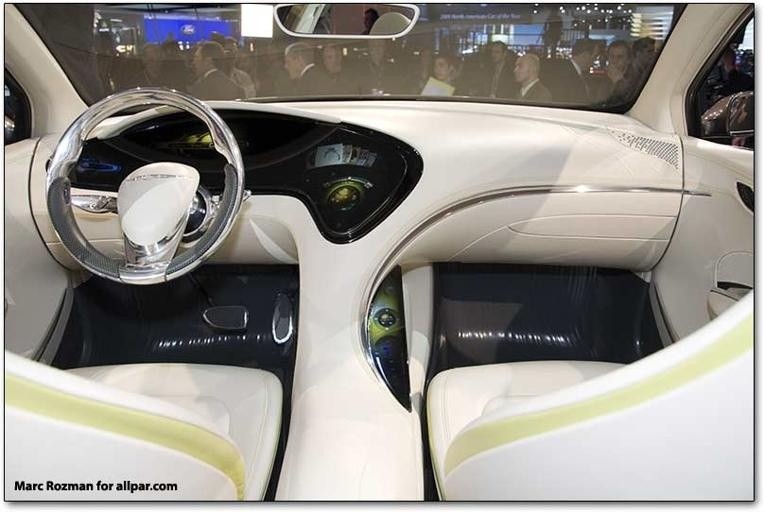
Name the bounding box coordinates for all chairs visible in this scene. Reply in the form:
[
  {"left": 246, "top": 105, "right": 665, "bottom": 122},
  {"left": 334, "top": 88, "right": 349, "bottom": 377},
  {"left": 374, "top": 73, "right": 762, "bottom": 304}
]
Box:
[{"left": 3, "top": 284, "right": 756, "bottom": 503}]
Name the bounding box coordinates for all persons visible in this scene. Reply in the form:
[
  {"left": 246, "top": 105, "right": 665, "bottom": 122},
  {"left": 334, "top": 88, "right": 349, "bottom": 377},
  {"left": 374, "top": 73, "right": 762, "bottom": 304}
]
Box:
[{"left": 56, "top": 6, "right": 755, "bottom": 152}]
